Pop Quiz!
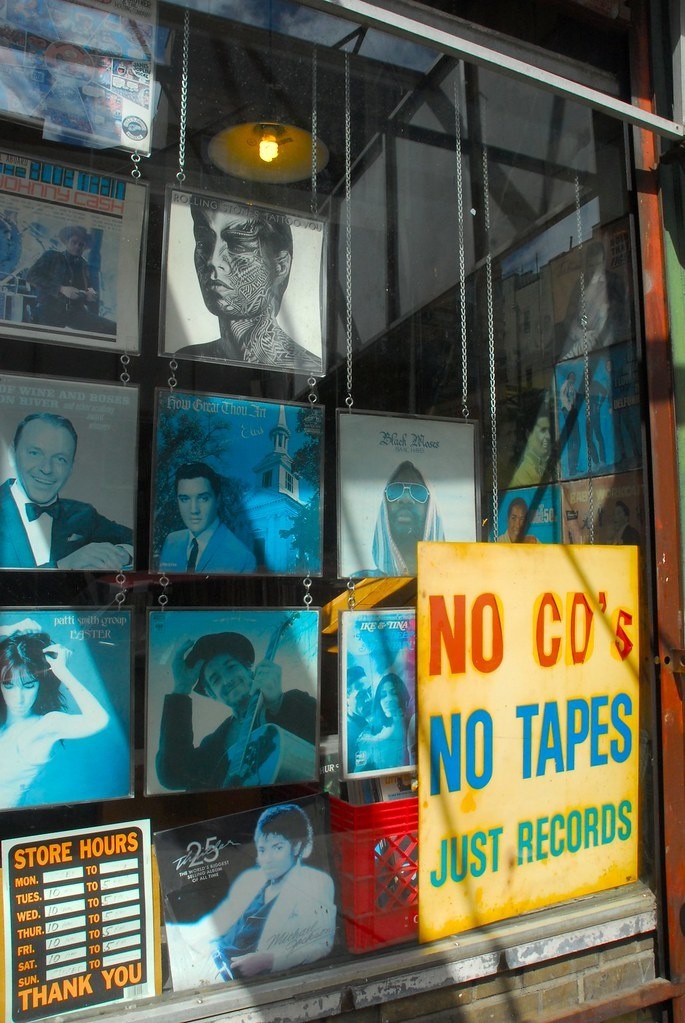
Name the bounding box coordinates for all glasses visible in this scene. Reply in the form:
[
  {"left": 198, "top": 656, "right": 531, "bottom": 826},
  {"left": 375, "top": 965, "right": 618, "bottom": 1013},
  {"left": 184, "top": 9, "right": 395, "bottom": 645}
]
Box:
[{"left": 385, "top": 483, "right": 429, "bottom": 504}]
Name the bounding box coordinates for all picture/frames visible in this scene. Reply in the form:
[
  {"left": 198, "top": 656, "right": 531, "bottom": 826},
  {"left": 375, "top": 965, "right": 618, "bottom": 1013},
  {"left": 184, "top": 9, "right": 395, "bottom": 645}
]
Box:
[
  {"left": 148, "top": 386, "right": 325, "bottom": 578},
  {"left": 157, "top": 182, "right": 331, "bottom": 378},
  {"left": 142, "top": 604, "right": 322, "bottom": 798},
  {"left": 335, "top": 405, "right": 483, "bottom": 580},
  {"left": 337, "top": 606, "right": 418, "bottom": 781},
  {"left": 0, "top": 371, "right": 141, "bottom": 573},
  {"left": 0, "top": 148, "right": 151, "bottom": 360},
  {"left": 0, "top": 0, "right": 161, "bottom": 160},
  {"left": 0, "top": 605, "right": 137, "bottom": 813}
]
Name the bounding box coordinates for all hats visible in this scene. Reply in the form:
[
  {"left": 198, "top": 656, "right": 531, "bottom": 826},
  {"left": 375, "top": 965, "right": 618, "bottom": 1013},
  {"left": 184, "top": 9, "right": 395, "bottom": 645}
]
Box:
[
  {"left": 59, "top": 226, "right": 94, "bottom": 250},
  {"left": 185, "top": 632, "right": 255, "bottom": 697}
]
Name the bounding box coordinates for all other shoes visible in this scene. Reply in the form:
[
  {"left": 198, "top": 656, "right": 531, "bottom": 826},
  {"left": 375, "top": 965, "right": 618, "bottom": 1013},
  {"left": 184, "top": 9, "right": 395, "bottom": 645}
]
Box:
[
  {"left": 588, "top": 461, "right": 607, "bottom": 474},
  {"left": 567, "top": 470, "right": 585, "bottom": 476},
  {"left": 613, "top": 461, "right": 625, "bottom": 472},
  {"left": 627, "top": 457, "right": 642, "bottom": 471}
]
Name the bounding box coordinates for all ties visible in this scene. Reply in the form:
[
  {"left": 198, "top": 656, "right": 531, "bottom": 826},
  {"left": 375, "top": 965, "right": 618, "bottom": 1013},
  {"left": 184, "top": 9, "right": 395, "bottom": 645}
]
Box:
[{"left": 186, "top": 538, "right": 199, "bottom": 574}]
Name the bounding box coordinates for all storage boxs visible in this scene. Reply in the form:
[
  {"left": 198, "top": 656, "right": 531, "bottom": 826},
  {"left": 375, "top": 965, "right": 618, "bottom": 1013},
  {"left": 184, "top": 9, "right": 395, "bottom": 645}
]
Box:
[{"left": 260, "top": 780, "right": 420, "bottom": 956}]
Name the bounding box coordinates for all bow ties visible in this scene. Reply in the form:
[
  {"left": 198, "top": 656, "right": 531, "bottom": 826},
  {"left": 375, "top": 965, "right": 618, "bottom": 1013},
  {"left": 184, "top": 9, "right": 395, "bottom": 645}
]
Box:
[{"left": 25, "top": 502, "right": 61, "bottom": 520}]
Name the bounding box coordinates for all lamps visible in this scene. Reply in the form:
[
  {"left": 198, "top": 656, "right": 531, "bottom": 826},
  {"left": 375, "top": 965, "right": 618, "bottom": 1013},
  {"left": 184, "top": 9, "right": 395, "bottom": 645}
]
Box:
[{"left": 206, "top": 1, "right": 330, "bottom": 184}]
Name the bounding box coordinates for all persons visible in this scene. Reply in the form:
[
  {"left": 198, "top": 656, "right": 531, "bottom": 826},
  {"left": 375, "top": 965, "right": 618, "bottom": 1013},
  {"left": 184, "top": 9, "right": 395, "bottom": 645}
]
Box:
[
  {"left": 183, "top": 803, "right": 338, "bottom": 978},
  {"left": 154, "top": 631, "right": 317, "bottom": 791},
  {"left": 347, "top": 665, "right": 415, "bottom": 774},
  {"left": 0, "top": 617, "right": 111, "bottom": 809},
  {"left": 496, "top": 497, "right": 541, "bottom": 543},
  {"left": 25, "top": 225, "right": 116, "bottom": 335},
  {"left": 498, "top": 405, "right": 553, "bottom": 492},
  {"left": 349, "top": 459, "right": 447, "bottom": 578},
  {"left": 174, "top": 193, "right": 322, "bottom": 374},
  {"left": 158, "top": 454, "right": 257, "bottom": 574},
  {"left": 612, "top": 501, "right": 641, "bottom": 545},
  {"left": 560, "top": 358, "right": 640, "bottom": 478},
  {"left": 0, "top": 411, "right": 134, "bottom": 570}
]
